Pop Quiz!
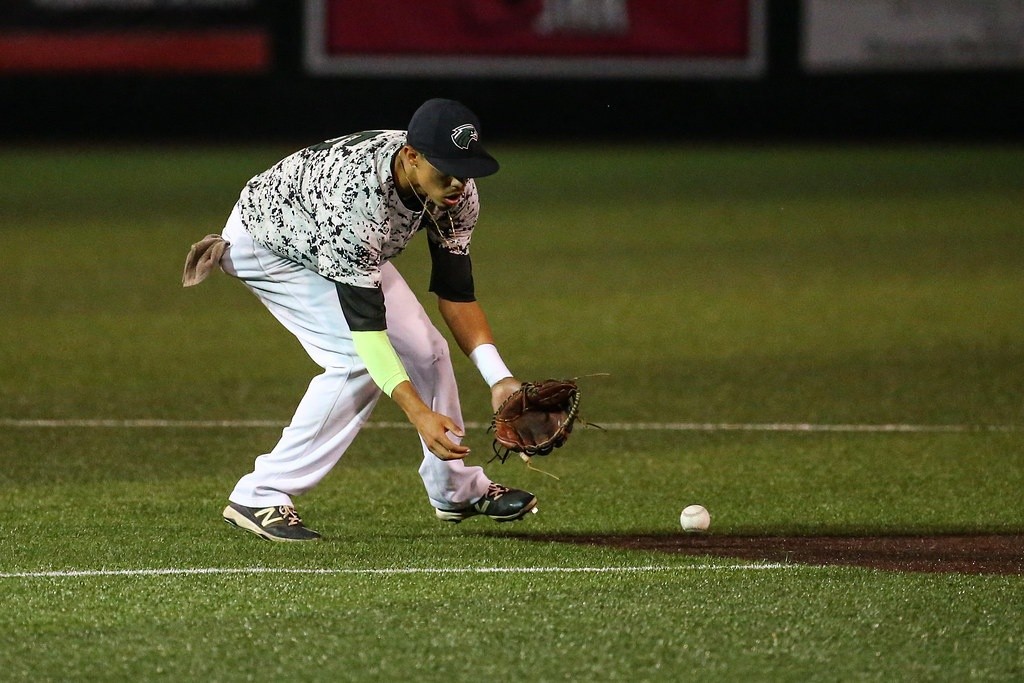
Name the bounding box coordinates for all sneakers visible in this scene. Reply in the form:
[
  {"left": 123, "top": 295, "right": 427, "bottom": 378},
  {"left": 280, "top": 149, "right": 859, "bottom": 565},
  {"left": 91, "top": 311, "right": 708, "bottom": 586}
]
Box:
[
  {"left": 435, "top": 480, "right": 538, "bottom": 524},
  {"left": 221, "top": 499, "right": 324, "bottom": 545}
]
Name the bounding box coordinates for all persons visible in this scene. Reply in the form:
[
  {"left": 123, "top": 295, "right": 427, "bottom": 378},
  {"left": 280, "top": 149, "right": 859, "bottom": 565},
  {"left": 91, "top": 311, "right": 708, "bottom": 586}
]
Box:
[{"left": 219, "top": 98, "right": 538, "bottom": 544}]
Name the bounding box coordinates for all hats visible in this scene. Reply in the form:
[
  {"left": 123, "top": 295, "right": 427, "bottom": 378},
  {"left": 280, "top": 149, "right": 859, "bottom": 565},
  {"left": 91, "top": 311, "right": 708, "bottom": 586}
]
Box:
[{"left": 405, "top": 96, "right": 499, "bottom": 178}]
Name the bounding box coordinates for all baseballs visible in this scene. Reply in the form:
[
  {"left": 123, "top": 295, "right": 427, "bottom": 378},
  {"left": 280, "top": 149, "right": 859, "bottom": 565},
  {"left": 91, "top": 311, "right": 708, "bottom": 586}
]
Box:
[{"left": 679, "top": 503, "right": 711, "bottom": 535}]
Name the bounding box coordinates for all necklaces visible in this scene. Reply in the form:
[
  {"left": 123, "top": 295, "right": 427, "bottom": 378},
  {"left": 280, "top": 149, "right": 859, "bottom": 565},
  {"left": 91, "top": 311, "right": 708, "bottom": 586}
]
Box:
[{"left": 399, "top": 149, "right": 459, "bottom": 252}]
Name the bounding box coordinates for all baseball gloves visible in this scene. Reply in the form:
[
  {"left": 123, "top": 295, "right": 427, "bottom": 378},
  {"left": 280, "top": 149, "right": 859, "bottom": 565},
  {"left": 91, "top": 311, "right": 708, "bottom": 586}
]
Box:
[{"left": 484, "top": 370, "right": 612, "bottom": 481}]
